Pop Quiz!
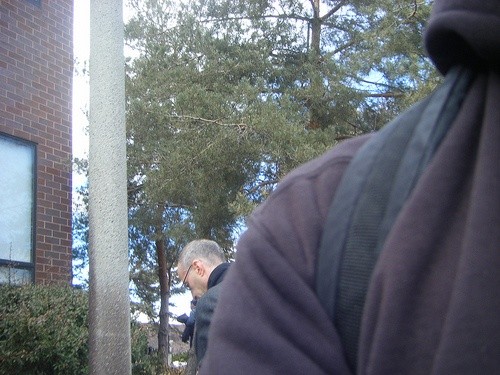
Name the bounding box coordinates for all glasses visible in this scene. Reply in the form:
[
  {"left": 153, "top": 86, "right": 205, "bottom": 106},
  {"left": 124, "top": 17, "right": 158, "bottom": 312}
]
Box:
[{"left": 182, "top": 264, "right": 194, "bottom": 290}]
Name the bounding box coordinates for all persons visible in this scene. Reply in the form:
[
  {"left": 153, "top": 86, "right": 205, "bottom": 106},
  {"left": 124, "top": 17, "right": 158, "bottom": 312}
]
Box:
[
  {"left": 178, "top": 239, "right": 232, "bottom": 368},
  {"left": 198, "top": 0, "right": 500, "bottom": 375},
  {"left": 186, "top": 300, "right": 196, "bottom": 348}
]
